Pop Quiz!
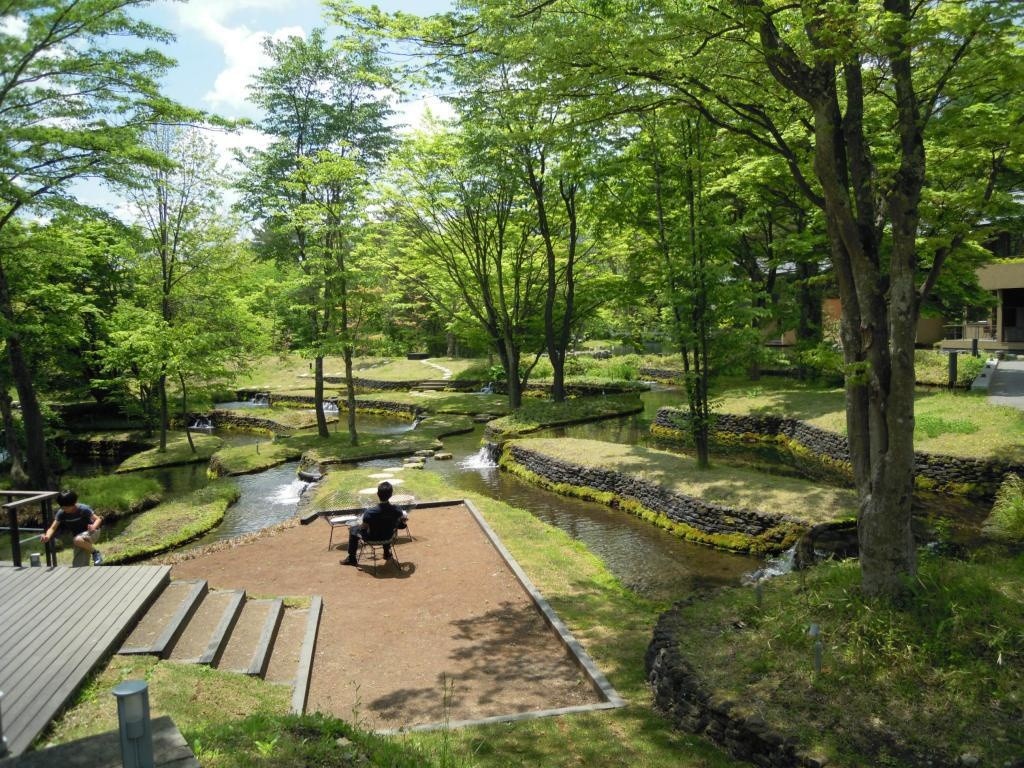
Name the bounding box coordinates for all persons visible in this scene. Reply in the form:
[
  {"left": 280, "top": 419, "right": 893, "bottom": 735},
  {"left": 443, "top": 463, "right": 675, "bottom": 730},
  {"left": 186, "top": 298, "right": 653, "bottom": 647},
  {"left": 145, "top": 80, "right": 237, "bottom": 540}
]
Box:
[
  {"left": 339, "top": 481, "right": 409, "bottom": 566},
  {"left": 39, "top": 488, "right": 103, "bottom": 567}
]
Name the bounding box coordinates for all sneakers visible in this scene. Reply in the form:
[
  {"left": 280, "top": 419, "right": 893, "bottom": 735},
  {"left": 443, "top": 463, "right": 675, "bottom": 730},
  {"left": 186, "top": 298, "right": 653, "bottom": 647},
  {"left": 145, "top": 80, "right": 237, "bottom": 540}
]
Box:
[{"left": 93, "top": 551, "right": 101, "bottom": 566}]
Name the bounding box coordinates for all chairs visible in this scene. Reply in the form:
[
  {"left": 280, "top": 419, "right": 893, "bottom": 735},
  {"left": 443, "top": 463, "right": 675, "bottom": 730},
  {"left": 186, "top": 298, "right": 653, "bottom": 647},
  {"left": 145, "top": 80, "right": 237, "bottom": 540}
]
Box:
[
  {"left": 320, "top": 509, "right": 359, "bottom": 552},
  {"left": 354, "top": 525, "right": 401, "bottom": 579},
  {"left": 388, "top": 501, "right": 417, "bottom": 544}
]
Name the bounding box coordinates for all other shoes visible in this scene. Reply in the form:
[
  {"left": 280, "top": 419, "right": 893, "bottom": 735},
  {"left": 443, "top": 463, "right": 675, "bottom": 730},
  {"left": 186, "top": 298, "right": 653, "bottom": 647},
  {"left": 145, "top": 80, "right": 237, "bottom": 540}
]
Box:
[
  {"left": 382, "top": 554, "right": 393, "bottom": 560},
  {"left": 340, "top": 557, "right": 357, "bottom": 566}
]
